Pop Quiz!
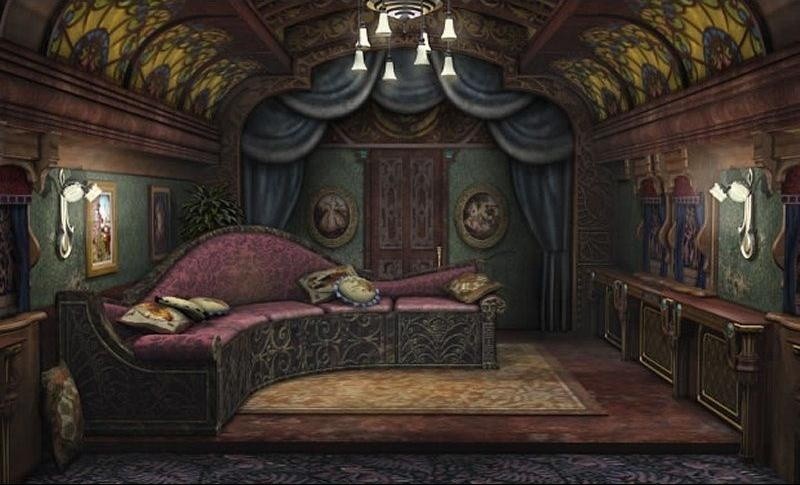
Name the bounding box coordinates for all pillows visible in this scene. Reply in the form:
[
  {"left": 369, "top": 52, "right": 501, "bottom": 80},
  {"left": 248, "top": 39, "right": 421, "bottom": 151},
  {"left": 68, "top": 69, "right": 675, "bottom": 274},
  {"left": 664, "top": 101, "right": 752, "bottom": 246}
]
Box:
[
  {"left": 116, "top": 300, "right": 193, "bottom": 334},
  {"left": 155, "top": 291, "right": 208, "bottom": 325},
  {"left": 440, "top": 267, "right": 506, "bottom": 303},
  {"left": 295, "top": 260, "right": 360, "bottom": 305},
  {"left": 330, "top": 273, "right": 384, "bottom": 308},
  {"left": 187, "top": 296, "right": 235, "bottom": 316}
]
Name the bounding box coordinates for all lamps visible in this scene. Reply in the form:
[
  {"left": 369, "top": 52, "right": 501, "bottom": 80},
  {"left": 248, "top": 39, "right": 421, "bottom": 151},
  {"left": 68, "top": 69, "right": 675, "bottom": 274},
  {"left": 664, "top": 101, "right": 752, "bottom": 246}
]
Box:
[
  {"left": 55, "top": 166, "right": 103, "bottom": 261},
  {"left": 708, "top": 164, "right": 757, "bottom": 261},
  {"left": 349, "top": 0, "right": 458, "bottom": 81}
]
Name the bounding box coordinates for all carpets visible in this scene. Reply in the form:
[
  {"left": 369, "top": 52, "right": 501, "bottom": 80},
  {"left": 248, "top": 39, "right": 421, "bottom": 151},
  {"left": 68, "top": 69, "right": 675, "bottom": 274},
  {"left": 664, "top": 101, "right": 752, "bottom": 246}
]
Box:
[{"left": 236, "top": 339, "right": 611, "bottom": 419}]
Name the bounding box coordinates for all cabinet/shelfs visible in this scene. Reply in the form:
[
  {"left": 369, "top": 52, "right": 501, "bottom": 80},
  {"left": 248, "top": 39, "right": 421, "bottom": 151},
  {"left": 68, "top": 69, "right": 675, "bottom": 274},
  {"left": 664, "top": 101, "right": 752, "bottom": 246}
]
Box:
[
  {"left": 0, "top": 310, "right": 52, "bottom": 485},
  {"left": 586, "top": 266, "right": 767, "bottom": 459}
]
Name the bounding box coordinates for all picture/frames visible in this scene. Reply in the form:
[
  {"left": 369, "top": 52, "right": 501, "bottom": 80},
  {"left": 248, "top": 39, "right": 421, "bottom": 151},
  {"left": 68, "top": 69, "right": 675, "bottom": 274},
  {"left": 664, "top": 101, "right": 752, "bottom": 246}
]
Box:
[
  {"left": 149, "top": 185, "right": 174, "bottom": 262},
  {"left": 304, "top": 184, "right": 361, "bottom": 251},
  {"left": 84, "top": 178, "right": 121, "bottom": 278},
  {"left": 452, "top": 181, "right": 511, "bottom": 251}
]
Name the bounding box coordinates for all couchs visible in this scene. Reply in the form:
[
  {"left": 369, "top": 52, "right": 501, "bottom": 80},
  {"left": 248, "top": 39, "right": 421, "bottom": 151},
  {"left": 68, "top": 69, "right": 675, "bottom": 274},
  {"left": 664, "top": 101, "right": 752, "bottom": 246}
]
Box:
[{"left": 54, "top": 220, "right": 509, "bottom": 439}]
